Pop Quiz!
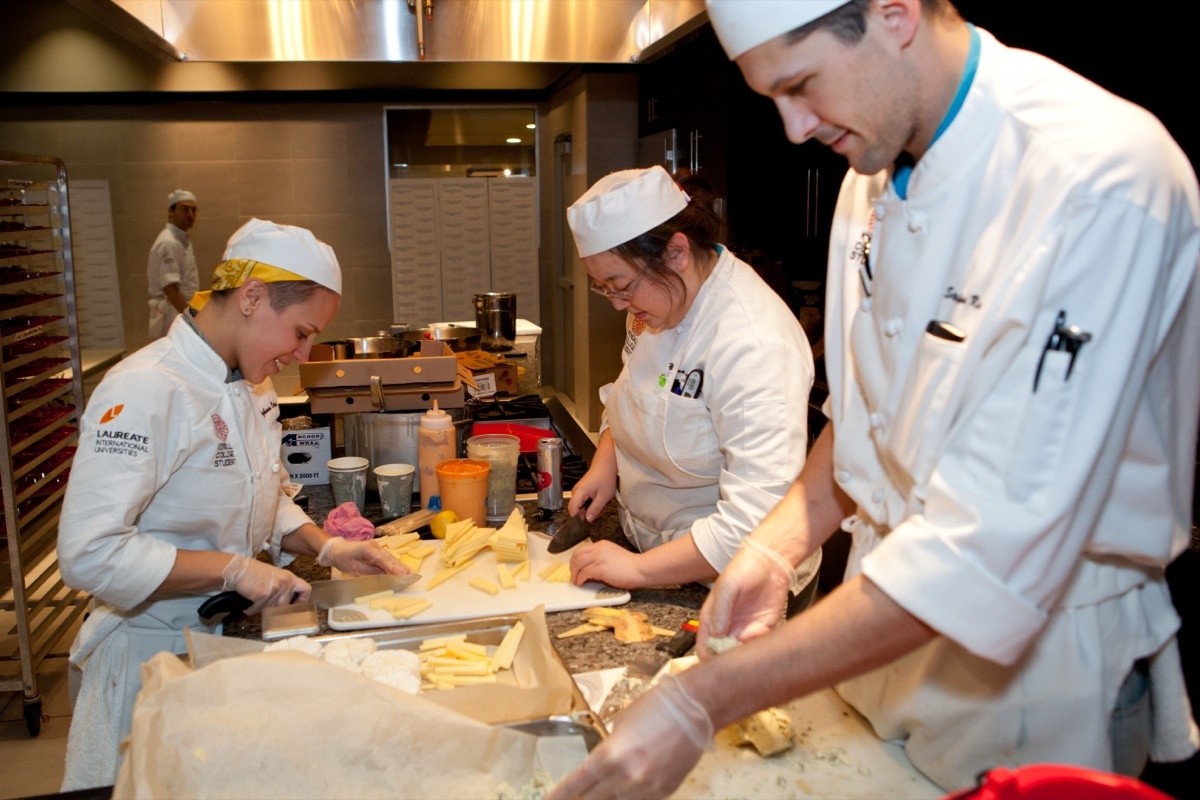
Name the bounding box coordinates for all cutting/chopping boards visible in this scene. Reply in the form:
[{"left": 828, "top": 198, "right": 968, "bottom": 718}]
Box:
[
  {"left": 570, "top": 655, "right": 948, "bottom": 800},
  {"left": 329, "top": 528, "right": 631, "bottom": 629}
]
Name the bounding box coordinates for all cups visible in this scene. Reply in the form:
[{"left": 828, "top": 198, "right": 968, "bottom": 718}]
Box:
[
  {"left": 326, "top": 455, "right": 370, "bottom": 514},
  {"left": 373, "top": 463, "right": 415, "bottom": 517},
  {"left": 467, "top": 434, "right": 521, "bottom": 524},
  {"left": 437, "top": 459, "right": 489, "bottom": 531}
]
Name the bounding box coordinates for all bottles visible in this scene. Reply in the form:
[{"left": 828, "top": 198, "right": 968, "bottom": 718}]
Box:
[{"left": 417, "top": 397, "right": 457, "bottom": 509}]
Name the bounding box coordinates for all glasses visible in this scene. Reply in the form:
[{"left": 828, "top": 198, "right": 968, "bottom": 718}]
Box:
[{"left": 590, "top": 257, "right": 657, "bottom": 300}]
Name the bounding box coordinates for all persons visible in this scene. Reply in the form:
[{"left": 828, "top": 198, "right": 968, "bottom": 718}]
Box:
[
  {"left": 145, "top": 189, "right": 200, "bottom": 340},
  {"left": 566, "top": 164, "right": 825, "bottom": 622},
  {"left": 55, "top": 217, "right": 415, "bottom": 792},
  {"left": 538, "top": 1, "right": 1200, "bottom": 799}
]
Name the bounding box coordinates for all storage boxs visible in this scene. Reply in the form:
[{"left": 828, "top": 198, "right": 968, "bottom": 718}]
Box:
[
  {"left": 279, "top": 422, "right": 332, "bottom": 486},
  {"left": 455, "top": 349, "right": 518, "bottom": 398},
  {"left": 299, "top": 338, "right": 456, "bottom": 389},
  {"left": 459, "top": 334, "right": 542, "bottom": 395},
  {"left": 307, "top": 381, "right": 466, "bottom": 414}
]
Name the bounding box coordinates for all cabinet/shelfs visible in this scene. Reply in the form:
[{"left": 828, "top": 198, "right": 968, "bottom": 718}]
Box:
[{"left": 0, "top": 155, "right": 96, "bottom": 738}]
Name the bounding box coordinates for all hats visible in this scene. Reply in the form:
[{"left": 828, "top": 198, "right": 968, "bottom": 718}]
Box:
[
  {"left": 705, "top": 0, "right": 851, "bottom": 63},
  {"left": 221, "top": 218, "right": 343, "bottom": 297},
  {"left": 566, "top": 164, "right": 693, "bottom": 260},
  {"left": 167, "top": 189, "right": 196, "bottom": 209}
]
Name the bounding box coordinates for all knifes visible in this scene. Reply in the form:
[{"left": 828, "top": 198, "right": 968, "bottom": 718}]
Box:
[
  {"left": 594, "top": 617, "right": 706, "bottom": 724},
  {"left": 197, "top": 571, "right": 420, "bottom": 619},
  {"left": 546, "top": 476, "right": 619, "bottom": 555}
]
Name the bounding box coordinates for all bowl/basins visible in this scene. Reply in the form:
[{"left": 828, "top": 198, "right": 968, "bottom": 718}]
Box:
[
  {"left": 317, "top": 291, "right": 517, "bottom": 361},
  {"left": 338, "top": 410, "right": 472, "bottom": 495}
]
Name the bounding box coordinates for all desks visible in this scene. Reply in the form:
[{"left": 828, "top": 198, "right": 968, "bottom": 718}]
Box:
[
  {"left": 220, "top": 494, "right": 949, "bottom": 799},
  {"left": 17, "top": 345, "right": 129, "bottom": 384}
]
